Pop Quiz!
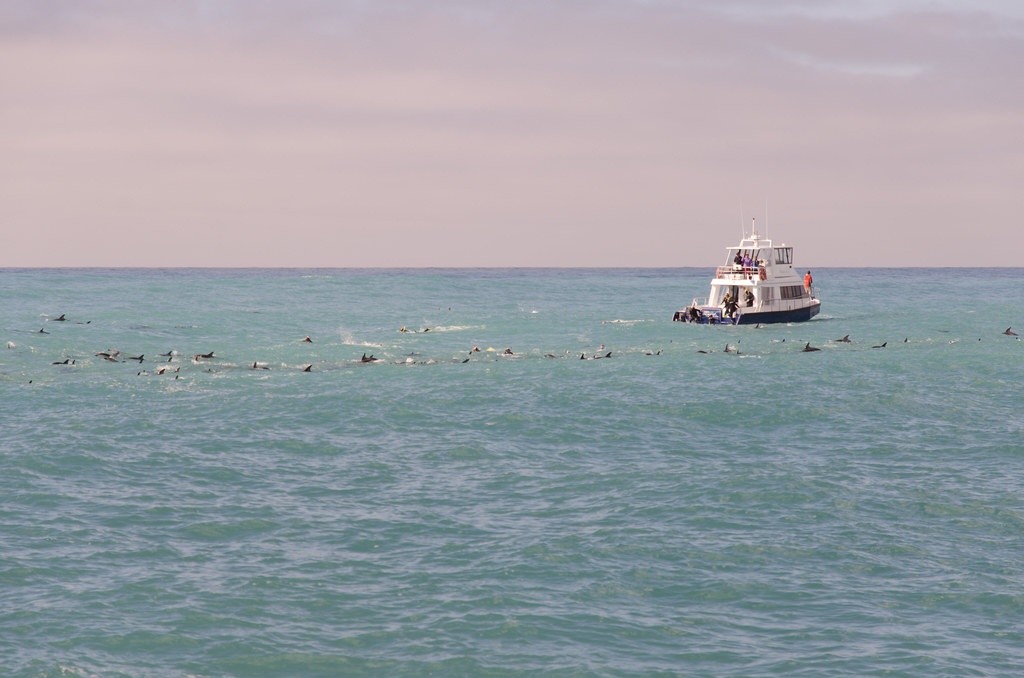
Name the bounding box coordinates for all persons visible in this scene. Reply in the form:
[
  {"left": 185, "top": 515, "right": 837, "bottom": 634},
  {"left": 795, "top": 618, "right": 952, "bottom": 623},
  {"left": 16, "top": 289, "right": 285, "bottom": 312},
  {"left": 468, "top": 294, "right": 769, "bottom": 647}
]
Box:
[
  {"left": 689, "top": 307, "right": 702, "bottom": 323},
  {"left": 734, "top": 252, "right": 743, "bottom": 274},
  {"left": 742, "top": 254, "right": 752, "bottom": 274},
  {"left": 804, "top": 270, "right": 812, "bottom": 288},
  {"left": 745, "top": 291, "right": 754, "bottom": 307},
  {"left": 721, "top": 293, "right": 739, "bottom": 321},
  {"left": 708, "top": 315, "right": 716, "bottom": 324}
]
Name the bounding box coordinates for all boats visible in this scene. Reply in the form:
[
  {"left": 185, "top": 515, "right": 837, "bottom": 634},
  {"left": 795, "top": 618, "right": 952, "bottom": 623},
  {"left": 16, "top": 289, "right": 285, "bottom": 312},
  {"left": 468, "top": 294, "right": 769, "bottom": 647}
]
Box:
[{"left": 682, "top": 217, "right": 821, "bottom": 324}]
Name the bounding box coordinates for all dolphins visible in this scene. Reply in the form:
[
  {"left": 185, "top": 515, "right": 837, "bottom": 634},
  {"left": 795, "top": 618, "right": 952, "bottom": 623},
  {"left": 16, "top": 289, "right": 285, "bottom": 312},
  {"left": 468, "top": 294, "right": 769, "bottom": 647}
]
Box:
[{"left": 27, "top": 313, "right": 1019, "bottom": 384}]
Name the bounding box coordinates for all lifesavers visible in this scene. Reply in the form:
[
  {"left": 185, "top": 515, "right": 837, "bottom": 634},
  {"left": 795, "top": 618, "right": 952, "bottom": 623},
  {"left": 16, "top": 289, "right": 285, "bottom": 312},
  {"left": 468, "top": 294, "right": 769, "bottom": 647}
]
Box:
[{"left": 759, "top": 267, "right": 767, "bottom": 281}]
